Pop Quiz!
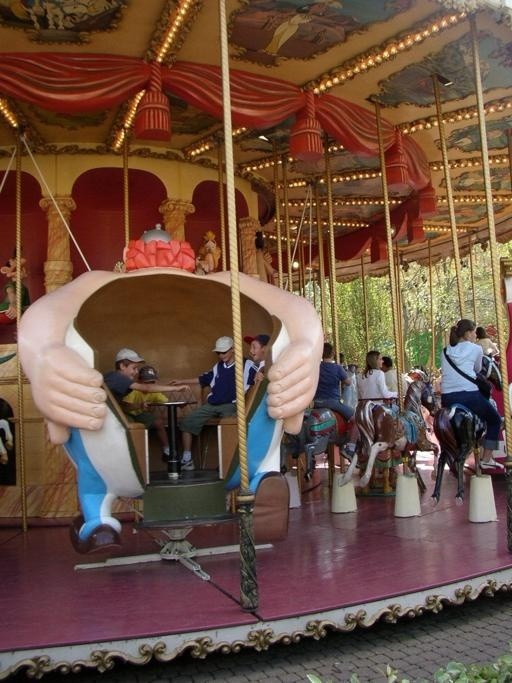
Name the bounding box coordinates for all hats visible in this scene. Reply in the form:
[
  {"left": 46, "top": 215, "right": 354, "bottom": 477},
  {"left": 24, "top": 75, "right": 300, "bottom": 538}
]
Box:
[
  {"left": 243, "top": 333, "right": 271, "bottom": 348},
  {"left": 211, "top": 335, "right": 235, "bottom": 353},
  {"left": 138, "top": 365, "right": 161, "bottom": 383},
  {"left": 116, "top": 347, "right": 148, "bottom": 366}
]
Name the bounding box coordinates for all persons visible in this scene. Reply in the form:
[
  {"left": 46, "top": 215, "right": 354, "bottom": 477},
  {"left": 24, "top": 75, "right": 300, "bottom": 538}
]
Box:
[
  {"left": 242, "top": 332, "right": 271, "bottom": 391},
  {"left": 167, "top": 335, "right": 255, "bottom": 470},
  {"left": 439, "top": 318, "right": 505, "bottom": 470},
  {"left": 312, "top": 341, "right": 413, "bottom": 419},
  {"left": 106, "top": 349, "right": 190, "bottom": 409},
  {"left": 473, "top": 325, "right": 501, "bottom": 369},
  {"left": 122, "top": 363, "right": 180, "bottom": 463}
]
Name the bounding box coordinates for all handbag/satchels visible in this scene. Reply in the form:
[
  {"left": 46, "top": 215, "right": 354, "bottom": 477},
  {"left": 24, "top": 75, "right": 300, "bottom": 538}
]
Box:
[{"left": 475, "top": 372, "right": 492, "bottom": 400}]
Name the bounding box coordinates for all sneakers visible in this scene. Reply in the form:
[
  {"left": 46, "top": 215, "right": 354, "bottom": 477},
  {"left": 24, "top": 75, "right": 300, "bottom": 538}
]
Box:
[
  {"left": 480, "top": 458, "right": 505, "bottom": 471},
  {"left": 162, "top": 453, "right": 195, "bottom": 471}
]
